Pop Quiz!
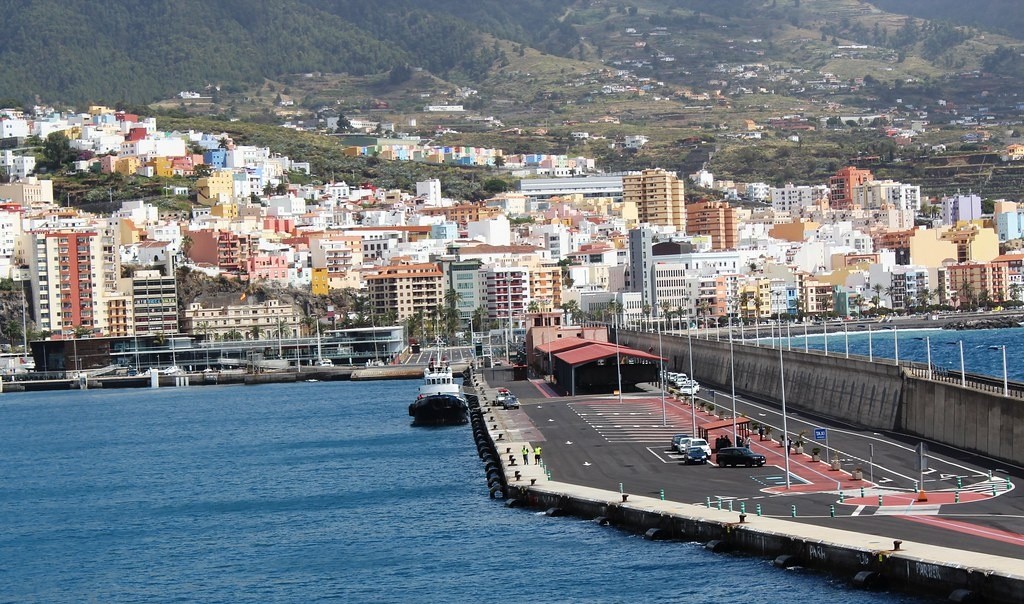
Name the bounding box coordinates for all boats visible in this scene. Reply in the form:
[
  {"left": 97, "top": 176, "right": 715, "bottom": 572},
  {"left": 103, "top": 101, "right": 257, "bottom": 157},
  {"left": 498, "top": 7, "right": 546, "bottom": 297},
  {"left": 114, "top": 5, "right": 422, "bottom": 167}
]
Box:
[
  {"left": 409, "top": 328, "right": 467, "bottom": 427},
  {"left": 314, "top": 358, "right": 334, "bottom": 368}
]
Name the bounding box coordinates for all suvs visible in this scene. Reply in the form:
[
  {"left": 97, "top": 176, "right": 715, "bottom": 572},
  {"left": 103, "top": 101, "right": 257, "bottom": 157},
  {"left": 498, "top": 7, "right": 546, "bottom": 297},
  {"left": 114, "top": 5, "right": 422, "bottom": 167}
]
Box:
[
  {"left": 716, "top": 447, "right": 767, "bottom": 468},
  {"left": 494, "top": 388, "right": 510, "bottom": 406}
]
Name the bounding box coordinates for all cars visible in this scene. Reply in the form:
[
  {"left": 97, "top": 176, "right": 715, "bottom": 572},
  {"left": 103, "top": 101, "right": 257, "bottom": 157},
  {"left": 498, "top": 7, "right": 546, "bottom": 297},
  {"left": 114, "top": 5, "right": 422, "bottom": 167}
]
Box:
[
  {"left": 671, "top": 434, "right": 711, "bottom": 465},
  {"left": 503, "top": 396, "right": 519, "bottom": 410},
  {"left": 660, "top": 370, "right": 700, "bottom": 395}
]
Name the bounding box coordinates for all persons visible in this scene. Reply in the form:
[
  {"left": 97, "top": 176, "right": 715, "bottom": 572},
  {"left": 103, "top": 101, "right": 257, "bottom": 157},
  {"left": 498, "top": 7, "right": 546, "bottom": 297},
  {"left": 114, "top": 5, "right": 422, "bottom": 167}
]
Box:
[
  {"left": 534, "top": 445, "right": 542, "bottom": 465},
  {"left": 720, "top": 434, "right": 731, "bottom": 448},
  {"left": 522, "top": 446, "right": 529, "bottom": 465},
  {"left": 759, "top": 427, "right": 764, "bottom": 441},
  {"left": 787, "top": 436, "right": 792, "bottom": 456},
  {"left": 745, "top": 435, "right": 751, "bottom": 449}
]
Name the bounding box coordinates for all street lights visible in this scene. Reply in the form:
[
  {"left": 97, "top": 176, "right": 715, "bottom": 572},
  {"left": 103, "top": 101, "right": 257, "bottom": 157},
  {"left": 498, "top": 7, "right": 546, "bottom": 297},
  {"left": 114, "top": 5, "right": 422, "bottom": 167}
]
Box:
[
  {"left": 612, "top": 300, "right": 622, "bottom": 403},
  {"left": 814, "top": 321, "right": 827, "bottom": 357},
  {"left": 987, "top": 346, "right": 1008, "bottom": 396},
  {"left": 944, "top": 339, "right": 966, "bottom": 388},
  {"left": 795, "top": 320, "right": 809, "bottom": 353},
  {"left": 654, "top": 303, "right": 666, "bottom": 425},
  {"left": 911, "top": 337, "right": 932, "bottom": 380},
  {"left": 542, "top": 299, "right": 552, "bottom": 382},
  {"left": 880, "top": 325, "right": 898, "bottom": 366},
  {"left": 768, "top": 289, "right": 790, "bottom": 489},
  {"left": 857, "top": 323, "right": 872, "bottom": 362},
  {"left": 832, "top": 323, "right": 849, "bottom": 360},
  {"left": 683, "top": 295, "right": 696, "bottom": 439}
]
